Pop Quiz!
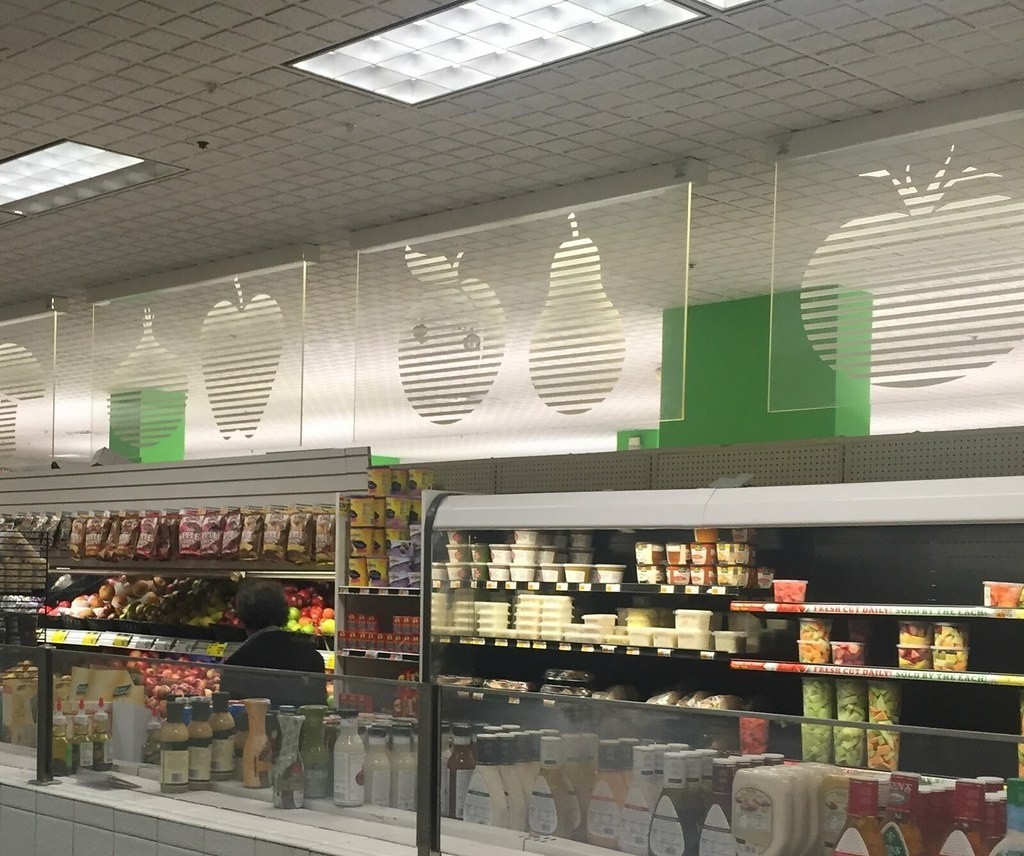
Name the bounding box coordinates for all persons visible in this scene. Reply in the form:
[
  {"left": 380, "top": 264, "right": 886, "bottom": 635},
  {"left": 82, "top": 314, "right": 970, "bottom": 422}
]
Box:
[{"left": 219, "top": 584, "right": 328, "bottom": 705}]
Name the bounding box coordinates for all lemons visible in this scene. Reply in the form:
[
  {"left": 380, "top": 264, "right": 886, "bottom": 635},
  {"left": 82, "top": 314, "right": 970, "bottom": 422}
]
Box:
[{"left": 178, "top": 594, "right": 229, "bottom": 627}]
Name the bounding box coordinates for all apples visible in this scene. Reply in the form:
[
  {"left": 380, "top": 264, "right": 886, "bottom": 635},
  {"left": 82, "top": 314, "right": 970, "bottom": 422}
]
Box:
[{"left": 219, "top": 582, "right": 335, "bottom": 632}]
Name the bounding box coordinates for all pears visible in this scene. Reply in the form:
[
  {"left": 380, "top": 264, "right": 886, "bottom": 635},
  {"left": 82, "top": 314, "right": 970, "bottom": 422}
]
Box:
[{"left": 67, "top": 576, "right": 169, "bottom": 619}]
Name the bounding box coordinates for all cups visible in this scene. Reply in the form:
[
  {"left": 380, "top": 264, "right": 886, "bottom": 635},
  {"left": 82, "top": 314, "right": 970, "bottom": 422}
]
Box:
[
  {"left": 835, "top": 679, "right": 866, "bottom": 722},
  {"left": 801, "top": 723, "right": 832, "bottom": 764},
  {"left": 801, "top": 676, "right": 832, "bottom": 719},
  {"left": 867, "top": 681, "right": 901, "bottom": 725},
  {"left": 833, "top": 725, "right": 865, "bottom": 768},
  {"left": 739, "top": 718, "right": 769, "bottom": 755},
  {"left": 866, "top": 729, "right": 900, "bottom": 770}
]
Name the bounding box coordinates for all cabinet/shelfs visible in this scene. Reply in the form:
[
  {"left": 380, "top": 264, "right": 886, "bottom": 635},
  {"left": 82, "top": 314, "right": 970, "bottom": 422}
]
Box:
[
  {"left": 337, "top": 586, "right": 420, "bottom": 663},
  {"left": 728, "top": 599, "right": 1024, "bottom": 784},
  {"left": 430, "top": 577, "right": 793, "bottom": 727}
]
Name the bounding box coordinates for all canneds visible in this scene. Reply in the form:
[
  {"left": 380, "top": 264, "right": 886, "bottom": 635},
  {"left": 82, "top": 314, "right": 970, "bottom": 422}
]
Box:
[{"left": 346, "top": 465, "right": 435, "bottom": 589}]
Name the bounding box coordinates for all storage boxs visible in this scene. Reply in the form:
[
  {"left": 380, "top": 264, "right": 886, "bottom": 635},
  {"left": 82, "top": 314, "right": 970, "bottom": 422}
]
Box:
[
  {"left": 626, "top": 607, "right": 658, "bottom": 619},
  {"left": 579, "top": 634, "right": 607, "bottom": 643},
  {"left": 540, "top": 621, "right": 565, "bottom": 632},
  {"left": 538, "top": 632, "right": 564, "bottom": 640},
  {"left": 713, "top": 630, "right": 748, "bottom": 652},
  {"left": 581, "top": 614, "right": 618, "bottom": 626},
  {"left": 539, "top": 613, "right": 575, "bottom": 623},
  {"left": 475, "top": 593, "right": 543, "bottom": 641},
  {"left": 338, "top": 614, "right": 419, "bottom": 719},
  {"left": 613, "top": 626, "right": 628, "bottom": 635},
  {"left": 562, "top": 633, "right": 581, "bottom": 642},
  {"left": 540, "top": 605, "right": 575, "bottom": 614},
  {"left": 625, "top": 618, "right": 659, "bottom": 627},
  {"left": 673, "top": 608, "right": 713, "bottom": 631},
  {"left": 674, "top": 630, "right": 715, "bottom": 649},
  {"left": 605, "top": 635, "right": 629, "bottom": 645},
  {"left": 563, "top": 623, "right": 584, "bottom": 633},
  {"left": 89, "top": 447, "right": 132, "bottom": 467},
  {"left": 652, "top": 627, "right": 678, "bottom": 648},
  {"left": 626, "top": 626, "right": 654, "bottom": 647},
  {"left": 581, "top": 624, "right": 616, "bottom": 636},
  {"left": 544, "top": 595, "right": 575, "bottom": 606}
]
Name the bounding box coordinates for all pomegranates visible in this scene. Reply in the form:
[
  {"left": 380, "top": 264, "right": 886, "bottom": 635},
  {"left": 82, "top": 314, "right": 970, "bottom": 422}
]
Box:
[{"left": 113, "top": 651, "right": 223, "bottom": 719}]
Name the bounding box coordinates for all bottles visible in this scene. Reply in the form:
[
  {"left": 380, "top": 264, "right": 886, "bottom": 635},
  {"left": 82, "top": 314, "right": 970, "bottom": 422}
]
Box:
[
  {"left": 52, "top": 701, "right": 72, "bottom": 775},
  {"left": 92, "top": 698, "right": 114, "bottom": 771},
  {"left": 440, "top": 721, "right": 784, "bottom": 856},
  {"left": 71, "top": 700, "right": 94, "bottom": 772},
  {"left": 142, "top": 692, "right": 418, "bottom": 811},
  {"left": 833, "top": 772, "right": 1024, "bottom": 855}
]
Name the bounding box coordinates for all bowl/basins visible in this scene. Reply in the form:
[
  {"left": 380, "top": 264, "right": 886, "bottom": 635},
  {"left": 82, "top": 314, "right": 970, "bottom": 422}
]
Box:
[
  {"left": 797, "top": 640, "right": 830, "bottom": 663},
  {"left": 432, "top": 593, "right": 476, "bottom": 636},
  {"left": 432, "top": 532, "right": 488, "bottom": 581},
  {"left": 930, "top": 646, "right": 970, "bottom": 671},
  {"left": 477, "top": 594, "right": 653, "bottom": 647},
  {"left": 982, "top": 581, "right": 1024, "bottom": 608},
  {"left": 674, "top": 608, "right": 712, "bottom": 631},
  {"left": 846, "top": 618, "right": 874, "bottom": 644},
  {"left": 799, "top": 618, "right": 833, "bottom": 640},
  {"left": 712, "top": 631, "right": 747, "bottom": 652},
  {"left": 932, "top": 622, "right": 969, "bottom": 647},
  {"left": 896, "top": 644, "right": 932, "bottom": 669},
  {"left": 830, "top": 641, "right": 865, "bottom": 664},
  {"left": 898, "top": 621, "right": 934, "bottom": 644},
  {"left": 771, "top": 580, "right": 808, "bottom": 602},
  {"left": 488, "top": 530, "right": 594, "bottom": 582},
  {"left": 653, "top": 627, "right": 676, "bottom": 648},
  {"left": 674, "top": 630, "right": 711, "bottom": 649},
  {"left": 595, "top": 564, "right": 627, "bottom": 583}
]
